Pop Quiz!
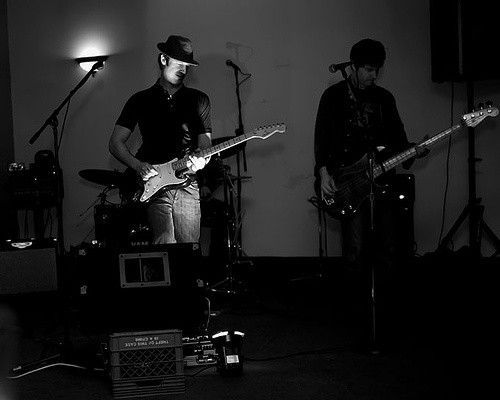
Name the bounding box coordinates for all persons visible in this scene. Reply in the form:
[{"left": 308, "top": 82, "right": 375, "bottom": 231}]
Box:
[
  {"left": 312, "top": 38, "right": 431, "bottom": 343},
  {"left": 107, "top": 34, "right": 215, "bottom": 245}
]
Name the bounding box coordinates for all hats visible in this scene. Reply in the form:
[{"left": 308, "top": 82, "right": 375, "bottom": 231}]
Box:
[{"left": 157, "top": 35, "right": 199, "bottom": 67}]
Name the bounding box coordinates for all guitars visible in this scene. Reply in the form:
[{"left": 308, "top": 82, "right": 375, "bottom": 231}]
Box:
[
  {"left": 322, "top": 100, "right": 500, "bottom": 223},
  {"left": 139, "top": 119, "right": 287, "bottom": 203}
]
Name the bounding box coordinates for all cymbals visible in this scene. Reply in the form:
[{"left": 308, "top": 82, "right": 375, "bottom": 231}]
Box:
[{"left": 79, "top": 168, "right": 140, "bottom": 193}]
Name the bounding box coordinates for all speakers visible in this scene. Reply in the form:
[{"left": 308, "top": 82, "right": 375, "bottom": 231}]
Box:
[{"left": 428, "top": 0, "right": 500, "bottom": 83}]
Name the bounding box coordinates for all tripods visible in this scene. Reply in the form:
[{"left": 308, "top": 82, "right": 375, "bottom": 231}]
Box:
[{"left": 432, "top": 85, "right": 500, "bottom": 258}]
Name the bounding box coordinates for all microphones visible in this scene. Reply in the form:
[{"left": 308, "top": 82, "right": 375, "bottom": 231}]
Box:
[
  {"left": 225, "top": 59, "right": 242, "bottom": 72},
  {"left": 329, "top": 61, "right": 356, "bottom": 73}
]
